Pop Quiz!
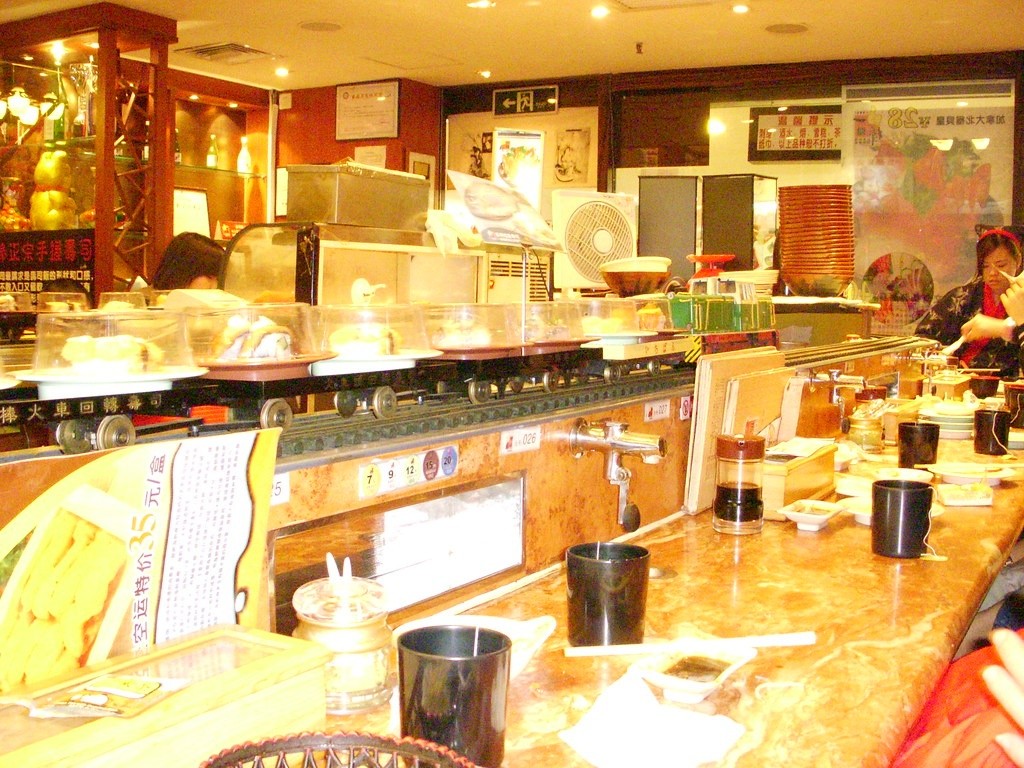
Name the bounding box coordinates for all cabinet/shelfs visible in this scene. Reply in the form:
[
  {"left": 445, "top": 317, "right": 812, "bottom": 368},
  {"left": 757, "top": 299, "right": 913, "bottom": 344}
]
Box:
[{"left": 1, "top": 1, "right": 178, "bottom": 309}]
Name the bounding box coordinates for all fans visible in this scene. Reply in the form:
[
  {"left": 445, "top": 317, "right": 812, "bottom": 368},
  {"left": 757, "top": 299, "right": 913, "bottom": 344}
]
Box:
[{"left": 553, "top": 189, "right": 638, "bottom": 299}]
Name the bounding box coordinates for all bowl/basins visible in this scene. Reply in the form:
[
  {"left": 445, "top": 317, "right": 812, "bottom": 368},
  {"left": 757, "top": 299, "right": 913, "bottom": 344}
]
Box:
[
  {"left": 916, "top": 401, "right": 976, "bottom": 441},
  {"left": 625, "top": 636, "right": 758, "bottom": 704},
  {"left": 971, "top": 432, "right": 1024, "bottom": 449},
  {"left": 836, "top": 495, "right": 945, "bottom": 527},
  {"left": 927, "top": 463, "right": 1015, "bottom": 486},
  {"left": 719, "top": 269, "right": 780, "bottom": 298},
  {"left": 601, "top": 271, "right": 672, "bottom": 298},
  {"left": 830, "top": 443, "right": 938, "bottom": 498},
  {"left": 778, "top": 184, "right": 856, "bottom": 298},
  {"left": 599, "top": 256, "right": 672, "bottom": 272},
  {"left": 778, "top": 498, "right": 845, "bottom": 534},
  {"left": 495, "top": 160, "right": 539, "bottom": 195}
]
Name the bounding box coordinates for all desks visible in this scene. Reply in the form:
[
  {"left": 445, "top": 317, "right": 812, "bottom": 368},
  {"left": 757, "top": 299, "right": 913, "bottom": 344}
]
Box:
[{"left": 300, "top": 423, "right": 1024, "bottom": 768}]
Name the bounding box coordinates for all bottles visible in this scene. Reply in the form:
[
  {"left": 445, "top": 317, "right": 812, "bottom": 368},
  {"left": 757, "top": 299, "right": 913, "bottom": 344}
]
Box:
[
  {"left": 711, "top": 433, "right": 766, "bottom": 535},
  {"left": 205, "top": 135, "right": 218, "bottom": 170},
  {"left": 174, "top": 128, "right": 181, "bottom": 164},
  {"left": 141, "top": 120, "right": 150, "bottom": 160},
  {"left": 236, "top": 136, "right": 251, "bottom": 178},
  {"left": 290, "top": 575, "right": 395, "bottom": 717}
]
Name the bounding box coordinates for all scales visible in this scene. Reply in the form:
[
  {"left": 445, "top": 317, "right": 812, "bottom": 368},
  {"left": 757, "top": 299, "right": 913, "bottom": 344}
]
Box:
[{"left": 685, "top": 254, "right": 736, "bottom": 291}]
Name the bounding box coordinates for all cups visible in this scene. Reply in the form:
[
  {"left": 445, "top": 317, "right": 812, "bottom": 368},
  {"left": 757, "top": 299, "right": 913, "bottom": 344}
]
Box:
[
  {"left": 397, "top": 625, "right": 512, "bottom": 768},
  {"left": 897, "top": 422, "right": 941, "bottom": 471},
  {"left": 974, "top": 410, "right": 1011, "bottom": 456},
  {"left": 1004, "top": 384, "right": 1024, "bottom": 430},
  {"left": 871, "top": 480, "right": 933, "bottom": 559},
  {"left": 566, "top": 542, "right": 651, "bottom": 648},
  {"left": 971, "top": 376, "right": 1001, "bottom": 399},
  {"left": 847, "top": 415, "right": 883, "bottom": 454}
]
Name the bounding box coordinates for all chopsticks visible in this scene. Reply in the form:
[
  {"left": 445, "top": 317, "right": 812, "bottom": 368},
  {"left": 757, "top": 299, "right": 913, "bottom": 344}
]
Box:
[
  {"left": 563, "top": 631, "right": 816, "bottom": 656},
  {"left": 913, "top": 463, "right": 1024, "bottom": 469}
]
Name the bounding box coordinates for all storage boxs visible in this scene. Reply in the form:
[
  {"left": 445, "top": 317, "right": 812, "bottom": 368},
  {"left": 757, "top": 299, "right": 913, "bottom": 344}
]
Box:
[{"left": 286, "top": 165, "right": 430, "bottom": 233}]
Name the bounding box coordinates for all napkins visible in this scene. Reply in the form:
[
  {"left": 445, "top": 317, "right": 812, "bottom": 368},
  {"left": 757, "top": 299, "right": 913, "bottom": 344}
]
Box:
[{"left": 557, "top": 670, "right": 746, "bottom": 768}]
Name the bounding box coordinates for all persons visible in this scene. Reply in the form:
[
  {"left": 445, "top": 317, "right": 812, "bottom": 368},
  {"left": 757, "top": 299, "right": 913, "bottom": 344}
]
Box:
[
  {"left": 982, "top": 629, "right": 1024, "bottom": 768},
  {"left": 41, "top": 278, "right": 90, "bottom": 311},
  {"left": 914, "top": 224, "right": 1024, "bottom": 377},
  {"left": 138, "top": 231, "right": 226, "bottom": 290}
]
